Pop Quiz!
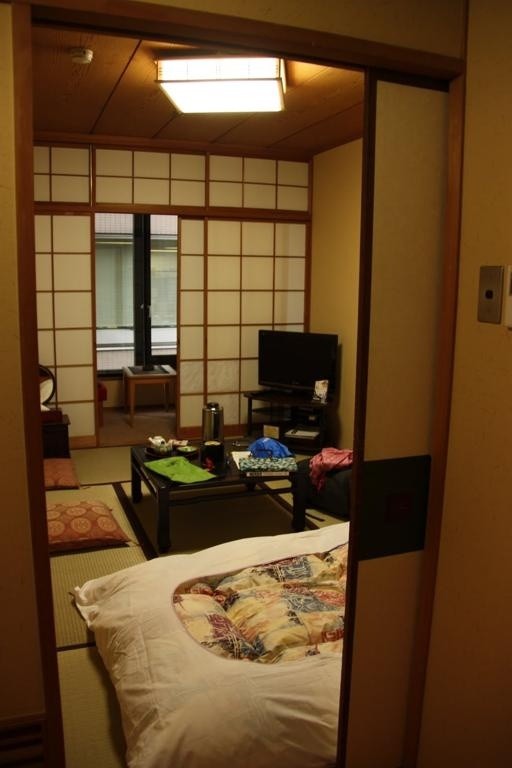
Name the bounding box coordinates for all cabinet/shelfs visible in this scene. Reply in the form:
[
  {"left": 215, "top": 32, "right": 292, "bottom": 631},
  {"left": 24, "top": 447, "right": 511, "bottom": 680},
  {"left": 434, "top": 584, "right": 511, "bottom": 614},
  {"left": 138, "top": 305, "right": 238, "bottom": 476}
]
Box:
[
  {"left": 41, "top": 415, "right": 71, "bottom": 458},
  {"left": 244, "top": 391, "right": 332, "bottom": 453}
]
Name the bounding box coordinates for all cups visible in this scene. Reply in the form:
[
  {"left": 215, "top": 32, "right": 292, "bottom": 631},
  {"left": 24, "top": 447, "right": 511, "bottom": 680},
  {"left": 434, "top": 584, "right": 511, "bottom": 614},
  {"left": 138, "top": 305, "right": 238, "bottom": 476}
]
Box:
[{"left": 202, "top": 440, "right": 220, "bottom": 471}]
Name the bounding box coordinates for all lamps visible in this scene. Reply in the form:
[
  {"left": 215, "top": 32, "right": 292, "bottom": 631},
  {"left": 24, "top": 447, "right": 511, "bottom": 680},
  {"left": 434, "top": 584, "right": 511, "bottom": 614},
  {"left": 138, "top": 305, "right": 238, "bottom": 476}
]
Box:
[{"left": 155, "top": 52, "right": 290, "bottom": 114}]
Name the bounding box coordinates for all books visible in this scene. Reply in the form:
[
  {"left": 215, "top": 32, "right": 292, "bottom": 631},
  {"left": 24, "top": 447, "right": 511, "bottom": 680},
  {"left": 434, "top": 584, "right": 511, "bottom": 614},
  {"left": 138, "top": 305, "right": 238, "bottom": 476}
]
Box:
[
  {"left": 286, "top": 430, "right": 319, "bottom": 439},
  {"left": 245, "top": 470, "right": 289, "bottom": 476}
]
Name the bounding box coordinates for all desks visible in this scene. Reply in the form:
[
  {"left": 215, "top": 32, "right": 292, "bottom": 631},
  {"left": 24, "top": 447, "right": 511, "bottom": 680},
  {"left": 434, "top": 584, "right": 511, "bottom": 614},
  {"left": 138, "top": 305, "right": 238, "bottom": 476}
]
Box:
[
  {"left": 130, "top": 439, "right": 307, "bottom": 553},
  {"left": 123, "top": 365, "right": 177, "bottom": 427}
]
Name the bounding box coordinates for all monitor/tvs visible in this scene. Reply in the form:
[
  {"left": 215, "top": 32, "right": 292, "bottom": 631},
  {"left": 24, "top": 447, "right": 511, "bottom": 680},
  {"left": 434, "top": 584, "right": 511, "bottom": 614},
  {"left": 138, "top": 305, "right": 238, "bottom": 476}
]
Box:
[{"left": 258, "top": 329, "right": 338, "bottom": 396}]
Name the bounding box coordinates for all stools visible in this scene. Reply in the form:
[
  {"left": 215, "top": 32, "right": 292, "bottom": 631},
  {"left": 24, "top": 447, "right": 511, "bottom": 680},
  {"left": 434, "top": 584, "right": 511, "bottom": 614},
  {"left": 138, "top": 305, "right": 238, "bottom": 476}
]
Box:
[
  {"left": 293, "top": 457, "right": 352, "bottom": 519},
  {"left": 98, "top": 383, "right": 107, "bottom": 427}
]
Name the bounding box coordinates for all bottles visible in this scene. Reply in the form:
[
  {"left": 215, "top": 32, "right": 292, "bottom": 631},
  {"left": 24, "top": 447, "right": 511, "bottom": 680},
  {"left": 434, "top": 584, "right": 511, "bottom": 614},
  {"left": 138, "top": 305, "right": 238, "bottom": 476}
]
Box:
[{"left": 202, "top": 402, "right": 225, "bottom": 442}]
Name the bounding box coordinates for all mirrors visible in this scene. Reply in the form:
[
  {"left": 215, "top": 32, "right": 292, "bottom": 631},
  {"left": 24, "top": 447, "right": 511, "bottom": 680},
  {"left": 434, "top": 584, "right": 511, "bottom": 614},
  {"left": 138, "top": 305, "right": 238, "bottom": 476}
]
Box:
[{"left": 38, "top": 364, "right": 56, "bottom": 411}]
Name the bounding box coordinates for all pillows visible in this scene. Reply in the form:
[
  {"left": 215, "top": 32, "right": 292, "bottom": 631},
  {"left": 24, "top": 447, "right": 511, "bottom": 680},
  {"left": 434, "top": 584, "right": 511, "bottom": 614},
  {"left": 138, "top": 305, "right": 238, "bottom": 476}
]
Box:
[
  {"left": 43, "top": 458, "right": 80, "bottom": 490},
  {"left": 46, "top": 500, "right": 131, "bottom": 553},
  {"left": 73, "top": 521, "right": 351, "bottom": 767}
]
